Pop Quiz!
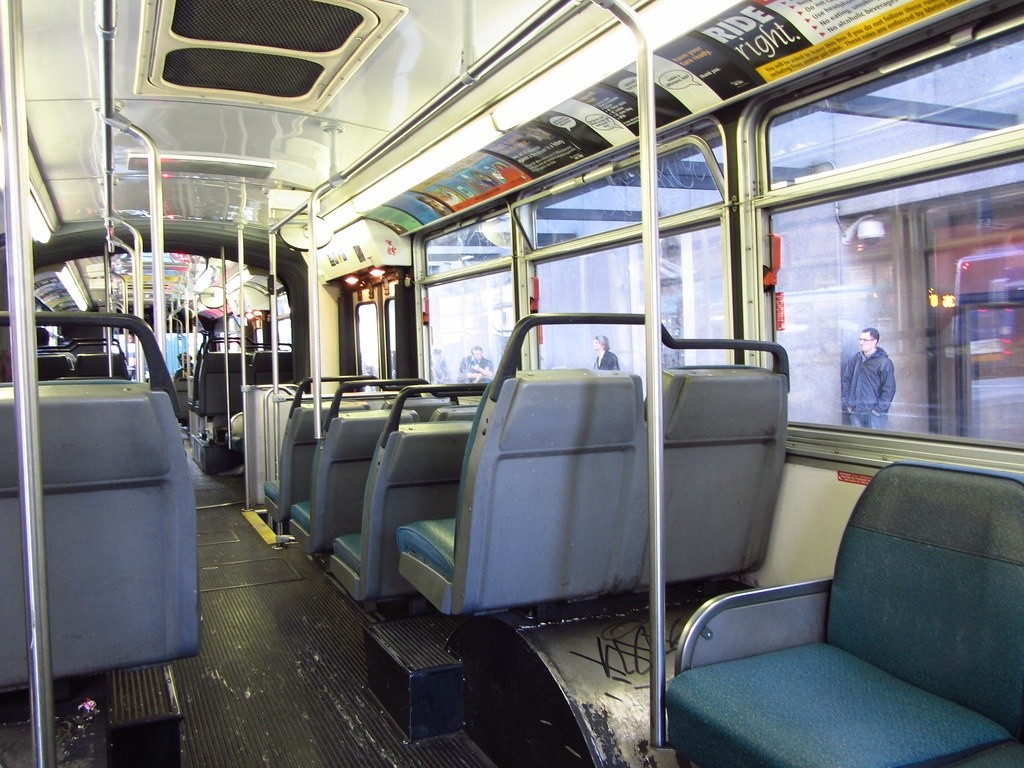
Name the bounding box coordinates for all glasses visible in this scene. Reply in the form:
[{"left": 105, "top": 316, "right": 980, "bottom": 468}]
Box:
[{"left": 859, "top": 338, "right": 874, "bottom": 342}]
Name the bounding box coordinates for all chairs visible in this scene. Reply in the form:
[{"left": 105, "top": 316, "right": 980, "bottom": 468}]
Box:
[
  {"left": 188, "top": 338, "right": 293, "bottom": 475},
  {"left": 662, "top": 456, "right": 1024, "bottom": 768},
  {"left": 262, "top": 309, "right": 790, "bottom": 620},
  {"left": 0, "top": 309, "right": 203, "bottom": 693}
]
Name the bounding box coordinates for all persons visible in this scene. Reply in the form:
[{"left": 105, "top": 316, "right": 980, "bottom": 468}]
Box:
[
  {"left": 592, "top": 335, "right": 620, "bottom": 370},
  {"left": 37, "top": 327, "right": 75, "bottom": 359},
  {"left": 430, "top": 345, "right": 494, "bottom": 384},
  {"left": 174, "top": 352, "right": 193, "bottom": 381},
  {"left": 128, "top": 333, "right": 134, "bottom": 342},
  {"left": 842, "top": 328, "right": 896, "bottom": 430}
]
